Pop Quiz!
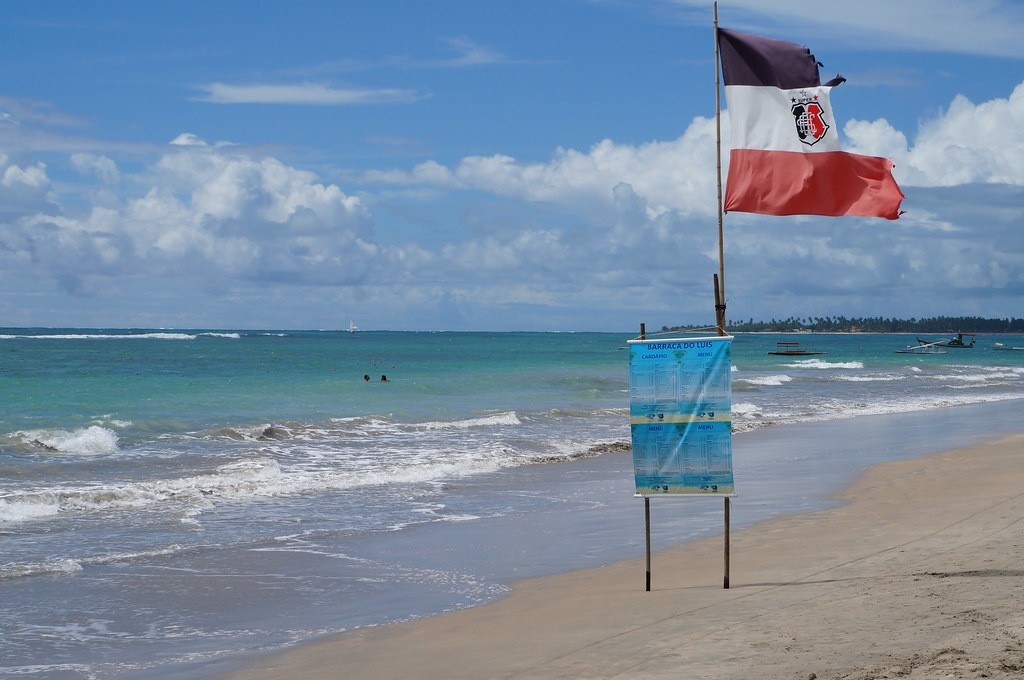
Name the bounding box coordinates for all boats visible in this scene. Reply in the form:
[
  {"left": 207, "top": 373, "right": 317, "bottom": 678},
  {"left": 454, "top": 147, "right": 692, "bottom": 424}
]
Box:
[
  {"left": 895, "top": 344, "right": 948, "bottom": 354},
  {"left": 768, "top": 343, "right": 828, "bottom": 355},
  {"left": 992, "top": 343, "right": 1024, "bottom": 351},
  {"left": 915, "top": 331, "right": 976, "bottom": 348}
]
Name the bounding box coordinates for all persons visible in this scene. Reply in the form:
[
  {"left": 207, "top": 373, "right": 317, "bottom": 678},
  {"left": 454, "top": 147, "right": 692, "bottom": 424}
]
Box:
[
  {"left": 381, "top": 375, "right": 386, "bottom": 380},
  {"left": 364, "top": 375, "right": 370, "bottom": 382}
]
